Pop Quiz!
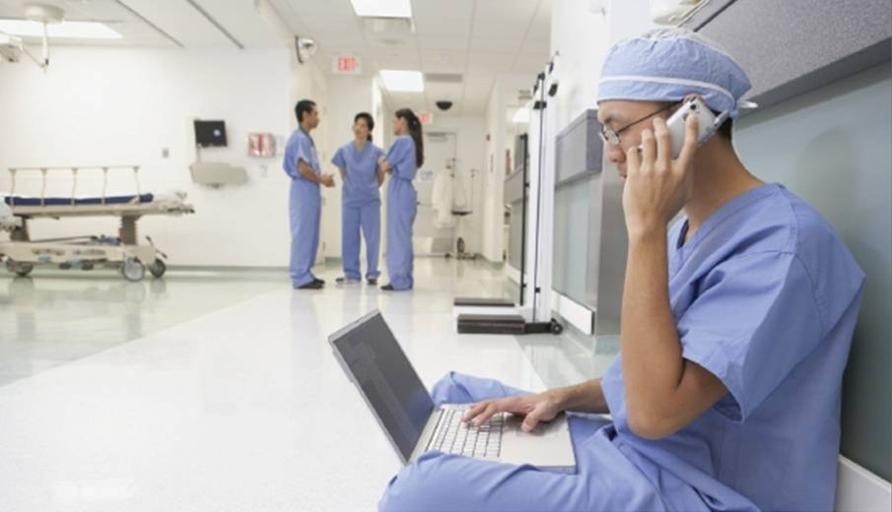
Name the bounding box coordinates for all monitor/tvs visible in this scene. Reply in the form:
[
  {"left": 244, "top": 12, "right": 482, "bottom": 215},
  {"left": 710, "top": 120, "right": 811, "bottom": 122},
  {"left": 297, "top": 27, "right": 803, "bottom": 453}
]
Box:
[{"left": 194, "top": 119, "right": 230, "bottom": 148}]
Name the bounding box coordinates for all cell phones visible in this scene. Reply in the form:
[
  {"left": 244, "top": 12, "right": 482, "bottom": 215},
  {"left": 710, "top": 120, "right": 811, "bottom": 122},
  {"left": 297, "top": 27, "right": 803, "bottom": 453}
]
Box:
[{"left": 638, "top": 96, "right": 729, "bottom": 162}]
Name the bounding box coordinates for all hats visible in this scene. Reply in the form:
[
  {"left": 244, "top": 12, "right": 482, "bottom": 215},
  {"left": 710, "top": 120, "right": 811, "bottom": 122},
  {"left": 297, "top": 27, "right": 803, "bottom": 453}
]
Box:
[{"left": 596, "top": 28, "right": 758, "bottom": 119}]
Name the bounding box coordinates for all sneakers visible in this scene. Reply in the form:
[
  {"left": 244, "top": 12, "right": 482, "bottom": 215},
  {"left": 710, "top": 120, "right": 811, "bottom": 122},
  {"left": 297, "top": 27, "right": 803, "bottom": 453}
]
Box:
[
  {"left": 313, "top": 278, "right": 324, "bottom": 284},
  {"left": 335, "top": 277, "right": 360, "bottom": 284},
  {"left": 367, "top": 279, "right": 377, "bottom": 287},
  {"left": 381, "top": 284, "right": 394, "bottom": 290},
  {"left": 299, "top": 280, "right": 322, "bottom": 289}
]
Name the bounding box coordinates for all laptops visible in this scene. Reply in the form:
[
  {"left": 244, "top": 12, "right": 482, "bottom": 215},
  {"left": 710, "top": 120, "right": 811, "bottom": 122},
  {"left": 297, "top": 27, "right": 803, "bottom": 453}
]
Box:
[{"left": 327, "top": 308, "right": 577, "bottom": 475}]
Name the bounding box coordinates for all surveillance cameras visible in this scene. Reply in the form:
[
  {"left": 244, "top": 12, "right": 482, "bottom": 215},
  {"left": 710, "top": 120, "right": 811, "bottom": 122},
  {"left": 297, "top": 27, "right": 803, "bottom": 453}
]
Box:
[
  {"left": 294, "top": 33, "right": 318, "bottom": 65},
  {"left": 436, "top": 101, "right": 454, "bottom": 112}
]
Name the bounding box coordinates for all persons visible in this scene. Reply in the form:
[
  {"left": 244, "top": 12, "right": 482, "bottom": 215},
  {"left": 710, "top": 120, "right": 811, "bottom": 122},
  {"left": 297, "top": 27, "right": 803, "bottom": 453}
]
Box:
[
  {"left": 380, "top": 25, "right": 865, "bottom": 512},
  {"left": 330, "top": 111, "right": 385, "bottom": 284},
  {"left": 282, "top": 98, "right": 337, "bottom": 290},
  {"left": 377, "top": 107, "right": 424, "bottom": 291}
]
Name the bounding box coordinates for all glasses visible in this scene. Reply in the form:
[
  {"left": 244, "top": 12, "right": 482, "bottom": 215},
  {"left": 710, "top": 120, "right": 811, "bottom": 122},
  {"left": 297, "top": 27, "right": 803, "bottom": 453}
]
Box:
[{"left": 598, "top": 100, "right": 681, "bottom": 147}]
